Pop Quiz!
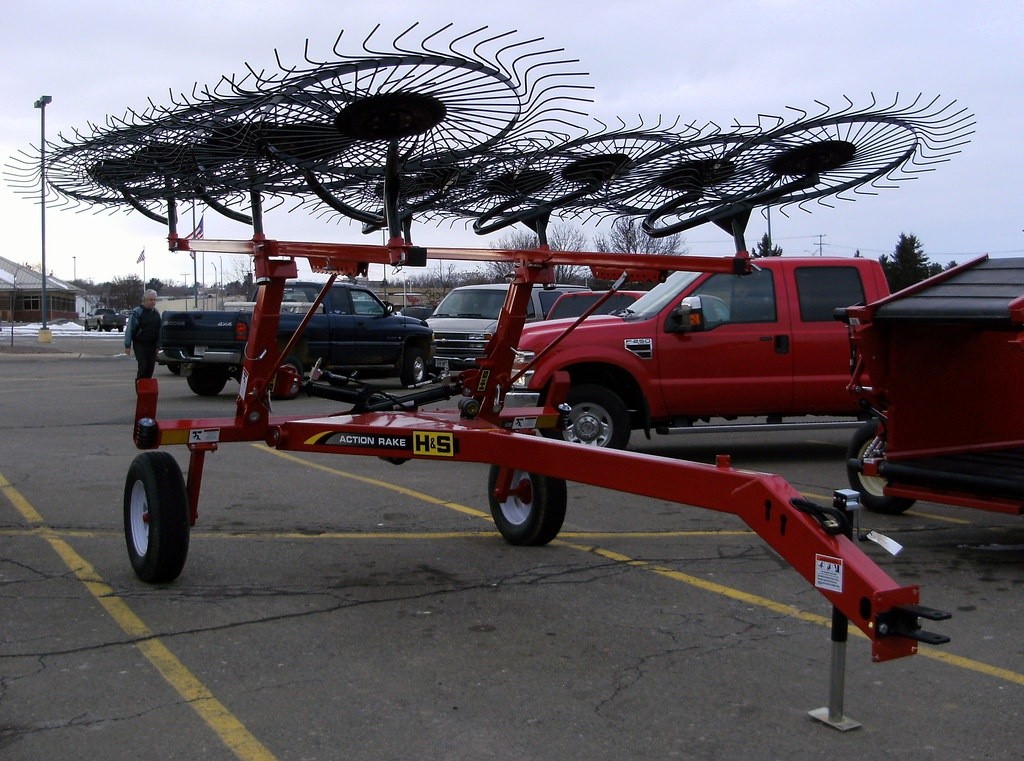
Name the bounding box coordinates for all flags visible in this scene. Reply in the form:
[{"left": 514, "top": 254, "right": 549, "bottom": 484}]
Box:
[
  {"left": 136, "top": 250, "right": 145, "bottom": 264},
  {"left": 185, "top": 214, "right": 203, "bottom": 240}
]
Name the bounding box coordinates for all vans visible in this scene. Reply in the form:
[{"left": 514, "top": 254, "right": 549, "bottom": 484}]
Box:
[{"left": 424, "top": 281, "right": 593, "bottom": 377}]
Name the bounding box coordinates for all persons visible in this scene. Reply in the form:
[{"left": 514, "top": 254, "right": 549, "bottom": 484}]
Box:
[{"left": 125, "top": 289, "right": 163, "bottom": 396}]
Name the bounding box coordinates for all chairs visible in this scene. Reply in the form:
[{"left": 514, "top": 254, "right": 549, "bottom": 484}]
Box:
[{"left": 735, "top": 277, "right": 773, "bottom": 322}]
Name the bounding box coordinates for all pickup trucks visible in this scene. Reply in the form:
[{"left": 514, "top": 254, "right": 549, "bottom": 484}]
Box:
[
  {"left": 156, "top": 280, "right": 436, "bottom": 397},
  {"left": 84, "top": 309, "right": 126, "bottom": 332},
  {"left": 506, "top": 257, "right": 894, "bottom": 450}
]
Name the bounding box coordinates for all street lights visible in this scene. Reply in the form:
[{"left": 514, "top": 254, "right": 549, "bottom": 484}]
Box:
[{"left": 34, "top": 96, "right": 52, "bottom": 343}]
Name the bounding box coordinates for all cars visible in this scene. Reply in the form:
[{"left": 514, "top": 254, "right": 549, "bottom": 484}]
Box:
[{"left": 545, "top": 290, "right": 651, "bottom": 321}]
